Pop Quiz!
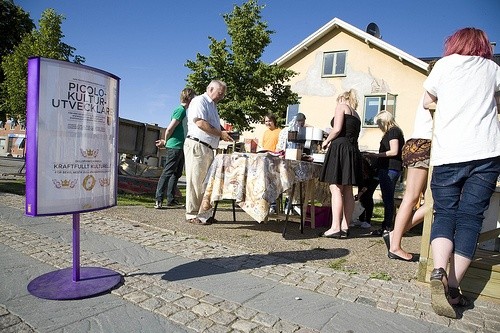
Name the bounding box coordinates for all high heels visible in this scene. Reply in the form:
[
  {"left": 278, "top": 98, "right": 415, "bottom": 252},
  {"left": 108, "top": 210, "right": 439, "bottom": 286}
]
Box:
[
  {"left": 318, "top": 229, "right": 341, "bottom": 240},
  {"left": 340, "top": 230, "right": 350, "bottom": 239}
]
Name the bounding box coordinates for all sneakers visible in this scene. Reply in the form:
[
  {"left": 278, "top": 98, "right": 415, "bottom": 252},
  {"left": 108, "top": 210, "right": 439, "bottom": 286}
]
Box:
[
  {"left": 349, "top": 220, "right": 361, "bottom": 227},
  {"left": 430, "top": 267, "right": 456, "bottom": 318},
  {"left": 154, "top": 201, "right": 163, "bottom": 209},
  {"left": 167, "top": 201, "right": 185, "bottom": 208},
  {"left": 445, "top": 287, "right": 470, "bottom": 307},
  {"left": 361, "top": 222, "right": 371, "bottom": 228}
]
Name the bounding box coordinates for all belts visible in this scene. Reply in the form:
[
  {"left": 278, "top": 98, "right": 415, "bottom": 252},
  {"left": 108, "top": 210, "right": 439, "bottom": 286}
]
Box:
[{"left": 186, "top": 135, "right": 214, "bottom": 150}]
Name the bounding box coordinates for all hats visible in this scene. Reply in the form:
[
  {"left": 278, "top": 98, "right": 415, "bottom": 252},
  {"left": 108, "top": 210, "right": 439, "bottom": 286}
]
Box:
[{"left": 295, "top": 113, "right": 306, "bottom": 121}]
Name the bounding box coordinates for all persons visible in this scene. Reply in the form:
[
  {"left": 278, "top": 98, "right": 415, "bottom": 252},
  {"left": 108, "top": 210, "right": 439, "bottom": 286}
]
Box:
[
  {"left": 154, "top": 87, "right": 195, "bottom": 209},
  {"left": 257, "top": 89, "right": 405, "bottom": 239},
  {"left": 183, "top": 80, "right": 234, "bottom": 225},
  {"left": 422, "top": 27, "right": 500, "bottom": 319},
  {"left": 383, "top": 57, "right": 440, "bottom": 262}
]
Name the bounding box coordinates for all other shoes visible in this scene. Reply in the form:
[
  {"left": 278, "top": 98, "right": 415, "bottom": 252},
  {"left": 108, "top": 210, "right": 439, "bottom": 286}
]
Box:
[
  {"left": 189, "top": 217, "right": 218, "bottom": 225},
  {"left": 285, "top": 208, "right": 294, "bottom": 215},
  {"left": 388, "top": 251, "right": 420, "bottom": 263},
  {"left": 271, "top": 205, "right": 277, "bottom": 214},
  {"left": 383, "top": 231, "right": 391, "bottom": 255},
  {"left": 371, "top": 228, "right": 387, "bottom": 237}
]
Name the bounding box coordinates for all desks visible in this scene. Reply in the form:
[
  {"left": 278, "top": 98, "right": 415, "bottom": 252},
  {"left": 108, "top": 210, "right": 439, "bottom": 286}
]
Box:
[
  {"left": 197, "top": 153, "right": 331, "bottom": 237},
  {"left": 417, "top": 104, "right": 500, "bottom": 300}
]
